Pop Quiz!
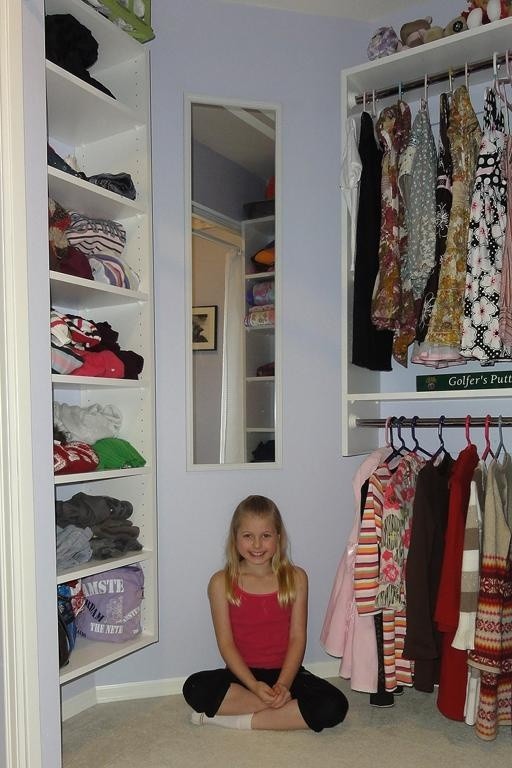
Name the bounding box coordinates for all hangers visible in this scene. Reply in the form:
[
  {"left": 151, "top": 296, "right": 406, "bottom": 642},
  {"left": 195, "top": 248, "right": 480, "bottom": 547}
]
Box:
[
  {"left": 377, "top": 416, "right": 510, "bottom": 463},
  {"left": 361, "top": 49, "right": 512, "bottom": 118}
]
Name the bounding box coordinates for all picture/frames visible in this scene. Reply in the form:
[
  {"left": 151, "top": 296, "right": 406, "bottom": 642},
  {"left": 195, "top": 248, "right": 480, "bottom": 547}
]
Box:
[{"left": 191, "top": 304, "right": 218, "bottom": 353}]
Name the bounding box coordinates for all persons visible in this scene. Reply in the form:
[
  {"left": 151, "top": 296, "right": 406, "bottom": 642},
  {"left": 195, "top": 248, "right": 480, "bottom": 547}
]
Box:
[{"left": 182, "top": 494, "right": 348, "bottom": 730}]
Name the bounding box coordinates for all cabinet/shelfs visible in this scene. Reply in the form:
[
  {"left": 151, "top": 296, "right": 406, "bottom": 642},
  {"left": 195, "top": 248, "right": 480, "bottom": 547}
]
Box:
[
  {"left": 23, "top": 0, "right": 161, "bottom": 684},
  {"left": 340, "top": 14, "right": 512, "bottom": 456},
  {"left": 237, "top": 214, "right": 276, "bottom": 464}
]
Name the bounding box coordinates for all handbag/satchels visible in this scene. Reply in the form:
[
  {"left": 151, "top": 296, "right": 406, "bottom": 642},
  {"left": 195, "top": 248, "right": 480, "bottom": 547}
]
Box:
[{"left": 74, "top": 565, "right": 144, "bottom": 644}]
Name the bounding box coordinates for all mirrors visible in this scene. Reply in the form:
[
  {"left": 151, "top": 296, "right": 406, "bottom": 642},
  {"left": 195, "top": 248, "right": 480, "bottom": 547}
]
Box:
[{"left": 183, "top": 90, "right": 283, "bottom": 474}]
